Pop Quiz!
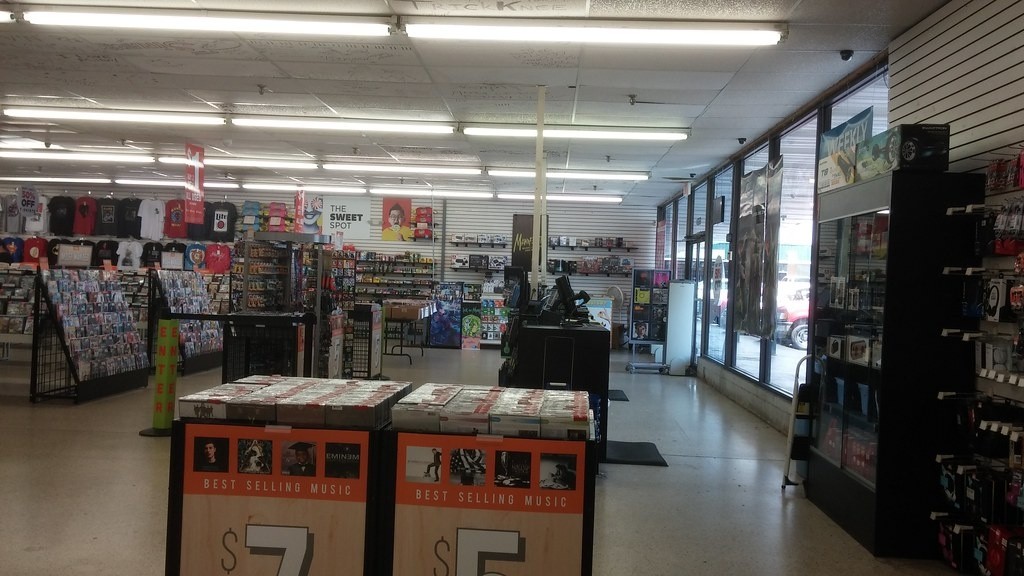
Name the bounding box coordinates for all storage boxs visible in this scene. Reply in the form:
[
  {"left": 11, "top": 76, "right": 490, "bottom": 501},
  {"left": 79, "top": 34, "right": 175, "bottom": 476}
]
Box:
[{"left": 938, "top": 150, "right": 1024, "bottom": 576}]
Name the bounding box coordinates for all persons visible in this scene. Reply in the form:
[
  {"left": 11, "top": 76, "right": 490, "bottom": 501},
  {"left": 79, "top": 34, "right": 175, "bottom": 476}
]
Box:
[
  {"left": 382, "top": 204, "right": 414, "bottom": 241},
  {"left": 635, "top": 323, "right": 648, "bottom": 340},
  {"left": 551, "top": 464, "right": 569, "bottom": 486},
  {"left": 424, "top": 449, "right": 442, "bottom": 482},
  {"left": 289, "top": 444, "right": 315, "bottom": 476},
  {"left": 199, "top": 443, "right": 223, "bottom": 471},
  {"left": 430, "top": 300, "right": 452, "bottom": 342}
]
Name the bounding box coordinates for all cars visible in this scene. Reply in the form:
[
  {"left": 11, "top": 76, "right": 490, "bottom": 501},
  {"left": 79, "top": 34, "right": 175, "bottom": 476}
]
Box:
[{"left": 697, "top": 270, "right": 810, "bottom": 351}]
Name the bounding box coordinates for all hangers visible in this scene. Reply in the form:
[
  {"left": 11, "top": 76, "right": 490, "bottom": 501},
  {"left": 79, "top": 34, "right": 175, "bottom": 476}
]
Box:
[
  {"left": 149, "top": 192, "right": 160, "bottom": 201},
  {"left": 101, "top": 193, "right": 115, "bottom": 199}
]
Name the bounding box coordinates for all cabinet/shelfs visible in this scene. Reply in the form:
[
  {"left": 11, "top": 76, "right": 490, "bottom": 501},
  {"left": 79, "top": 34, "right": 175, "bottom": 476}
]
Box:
[
  {"left": 407, "top": 222, "right": 439, "bottom": 242},
  {"left": 806, "top": 123, "right": 988, "bottom": 576},
  {"left": 0, "top": 240, "right": 639, "bottom": 576}
]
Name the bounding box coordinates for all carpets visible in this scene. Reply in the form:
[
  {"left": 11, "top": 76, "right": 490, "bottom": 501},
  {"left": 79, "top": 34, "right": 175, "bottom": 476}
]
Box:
[
  {"left": 607, "top": 390, "right": 629, "bottom": 401},
  {"left": 605, "top": 441, "right": 668, "bottom": 466}
]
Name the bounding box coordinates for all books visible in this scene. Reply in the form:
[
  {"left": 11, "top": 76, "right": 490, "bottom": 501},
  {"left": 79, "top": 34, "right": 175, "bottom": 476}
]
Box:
[
  {"left": 0, "top": 271, "right": 231, "bottom": 382},
  {"left": 178, "top": 375, "right": 590, "bottom": 440}
]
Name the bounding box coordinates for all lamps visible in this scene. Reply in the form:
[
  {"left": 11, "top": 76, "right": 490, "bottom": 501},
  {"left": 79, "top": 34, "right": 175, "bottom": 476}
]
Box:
[{"left": 0, "top": 0, "right": 790, "bottom": 205}]
